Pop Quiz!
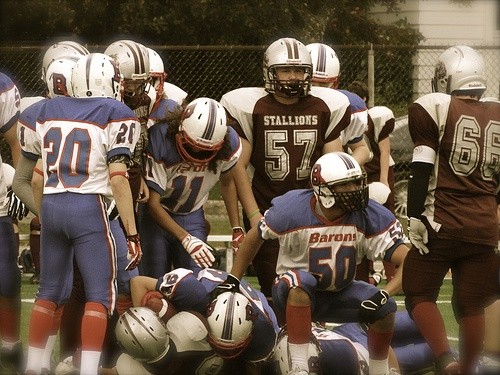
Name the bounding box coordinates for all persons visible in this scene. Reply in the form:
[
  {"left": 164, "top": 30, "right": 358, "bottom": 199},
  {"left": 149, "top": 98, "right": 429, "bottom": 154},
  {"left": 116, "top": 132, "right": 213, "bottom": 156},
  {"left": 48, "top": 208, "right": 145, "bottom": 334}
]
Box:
[
  {"left": 2, "top": 39, "right": 189, "bottom": 375},
  {"left": 346, "top": 80, "right": 403, "bottom": 295},
  {"left": 305, "top": 42, "right": 370, "bottom": 167},
  {"left": 402, "top": 45, "right": 500, "bottom": 375},
  {"left": 118, "top": 266, "right": 434, "bottom": 375},
  {"left": 143, "top": 96, "right": 265, "bottom": 276},
  {"left": 0, "top": 72, "right": 24, "bottom": 375},
  {"left": 211, "top": 151, "right": 411, "bottom": 375},
  {"left": 25, "top": 52, "right": 141, "bottom": 375},
  {"left": 220, "top": 37, "right": 352, "bottom": 306}
]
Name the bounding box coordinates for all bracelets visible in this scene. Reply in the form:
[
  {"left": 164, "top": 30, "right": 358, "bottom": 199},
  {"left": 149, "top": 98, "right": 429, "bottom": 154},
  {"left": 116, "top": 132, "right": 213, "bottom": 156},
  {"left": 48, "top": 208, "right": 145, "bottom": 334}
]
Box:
[{"left": 247, "top": 207, "right": 259, "bottom": 218}]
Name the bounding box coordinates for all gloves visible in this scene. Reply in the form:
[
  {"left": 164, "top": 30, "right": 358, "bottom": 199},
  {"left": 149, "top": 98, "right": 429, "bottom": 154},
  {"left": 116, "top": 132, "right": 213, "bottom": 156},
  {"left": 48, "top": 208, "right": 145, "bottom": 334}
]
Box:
[
  {"left": 4, "top": 186, "right": 29, "bottom": 220},
  {"left": 408, "top": 217, "right": 430, "bottom": 255},
  {"left": 359, "top": 289, "right": 390, "bottom": 324},
  {"left": 181, "top": 234, "right": 215, "bottom": 268},
  {"left": 125, "top": 233, "right": 144, "bottom": 271},
  {"left": 232, "top": 227, "right": 245, "bottom": 254},
  {"left": 210, "top": 274, "right": 241, "bottom": 302}
]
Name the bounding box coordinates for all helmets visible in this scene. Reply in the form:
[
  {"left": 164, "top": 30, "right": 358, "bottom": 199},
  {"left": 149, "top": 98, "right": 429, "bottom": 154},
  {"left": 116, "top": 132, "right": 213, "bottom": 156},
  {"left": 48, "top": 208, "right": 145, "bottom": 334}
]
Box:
[
  {"left": 176, "top": 97, "right": 227, "bottom": 166},
  {"left": 207, "top": 291, "right": 255, "bottom": 360},
  {"left": 305, "top": 43, "right": 341, "bottom": 89},
  {"left": 310, "top": 152, "right": 369, "bottom": 212},
  {"left": 434, "top": 45, "right": 488, "bottom": 95},
  {"left": 262, "top": 38, "right": 313, "bottom": 97},
  {"left": 274, "top": 331, "right": 321, "bottom": 375},
  {"left": 115, "top": 306, "right": 170, "bottom": 363},
  {"left": 40, "top": 39, "right": 165, "bottom": 105}
]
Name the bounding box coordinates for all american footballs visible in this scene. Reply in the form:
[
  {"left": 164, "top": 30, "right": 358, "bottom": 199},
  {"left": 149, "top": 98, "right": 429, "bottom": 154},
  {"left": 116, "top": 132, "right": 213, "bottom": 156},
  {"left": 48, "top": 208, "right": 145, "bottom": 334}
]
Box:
[{"left": 144, "top": 297, "right": 176, "bottom": 326}]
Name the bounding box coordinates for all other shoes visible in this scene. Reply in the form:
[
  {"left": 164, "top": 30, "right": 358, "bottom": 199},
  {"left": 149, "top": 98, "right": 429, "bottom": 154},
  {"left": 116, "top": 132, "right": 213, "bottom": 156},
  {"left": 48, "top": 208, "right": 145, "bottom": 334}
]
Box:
[
  {"left": 0, "top": 342, "right": 23, "bottom": 375},
  {"left": 30, "top": 273, "right": 40, "bottom": 283},
  {"left": 55, "top": 356, "right": 80, "bottom": 375},
  {"left": 436, "top": 356, "right": 459, "bottom": 375}
]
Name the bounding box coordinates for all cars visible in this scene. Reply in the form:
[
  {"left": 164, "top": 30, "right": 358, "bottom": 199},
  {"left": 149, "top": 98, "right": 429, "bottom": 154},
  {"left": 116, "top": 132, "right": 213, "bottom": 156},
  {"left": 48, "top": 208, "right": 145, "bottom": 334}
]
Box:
[{"left": 13, "top": 114, "right": 415, "bottom": 274}]
